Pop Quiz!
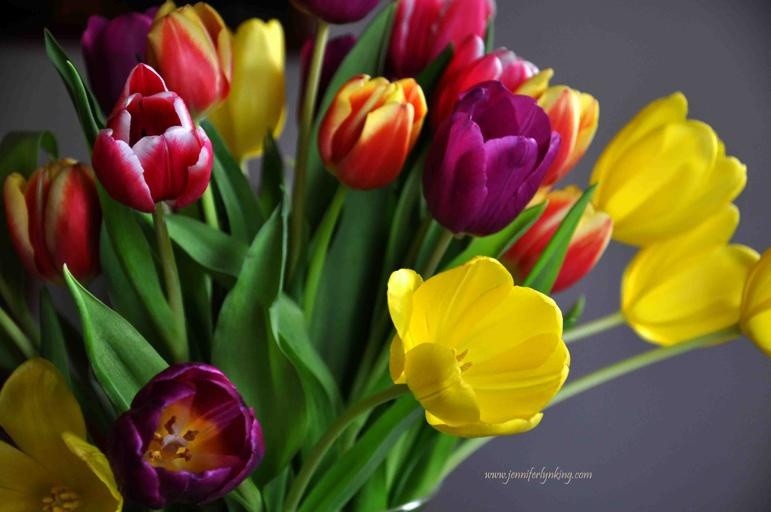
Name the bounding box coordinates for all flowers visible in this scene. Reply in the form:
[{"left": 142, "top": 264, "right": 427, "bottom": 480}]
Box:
[{"left": 0, "top": 0, "right": 771, "bottom": 510}]
